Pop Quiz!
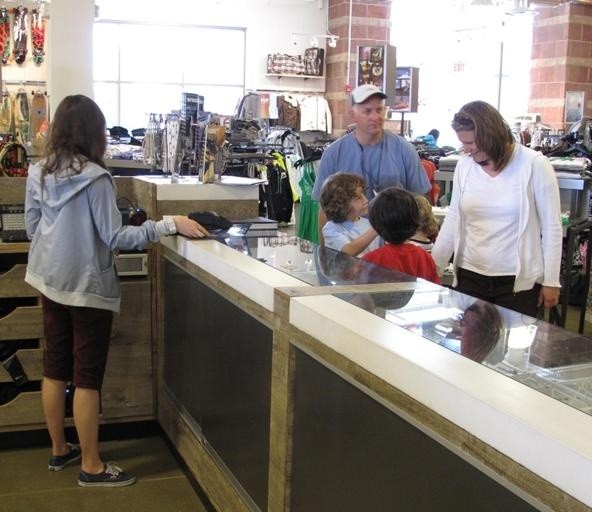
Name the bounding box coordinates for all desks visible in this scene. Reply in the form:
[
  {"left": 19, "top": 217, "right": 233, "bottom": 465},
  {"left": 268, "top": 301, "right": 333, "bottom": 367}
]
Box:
[{"left": 433, "top": 169, "right": 584, "bottom": 220}]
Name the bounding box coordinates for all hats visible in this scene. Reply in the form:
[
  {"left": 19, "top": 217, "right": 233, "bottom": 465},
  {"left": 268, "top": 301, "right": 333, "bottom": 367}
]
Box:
[{"left": 350, "top": 84, "right": 388, "bottom": 104}]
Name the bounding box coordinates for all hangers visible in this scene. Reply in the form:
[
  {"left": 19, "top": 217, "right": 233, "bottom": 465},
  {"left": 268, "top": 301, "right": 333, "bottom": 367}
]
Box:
[{"left": 293, "top": 143, "right": 324, "bottom": 170}]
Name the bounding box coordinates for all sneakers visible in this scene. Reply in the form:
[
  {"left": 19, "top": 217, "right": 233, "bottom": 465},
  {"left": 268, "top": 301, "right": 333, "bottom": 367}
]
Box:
[
  {"left": 78, "top": 465, "right": 135, "bottom": 488},
  {"left": 48, "top": 443, "right": 80, "bottom": 470}
]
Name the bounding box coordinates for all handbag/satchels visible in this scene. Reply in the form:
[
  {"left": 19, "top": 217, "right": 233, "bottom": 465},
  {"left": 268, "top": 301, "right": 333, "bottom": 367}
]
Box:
[
  {"left": 305, "top": 48, "right": 324, "bottom": 75},
  {"left": 269, "top": 52, "right": 306, "bottom": 75}
]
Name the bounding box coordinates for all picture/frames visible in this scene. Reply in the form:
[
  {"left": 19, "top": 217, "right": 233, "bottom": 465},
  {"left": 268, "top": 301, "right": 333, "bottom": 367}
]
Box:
[{"left": 564, "top": 91, "right": 585, "bottom": 125}]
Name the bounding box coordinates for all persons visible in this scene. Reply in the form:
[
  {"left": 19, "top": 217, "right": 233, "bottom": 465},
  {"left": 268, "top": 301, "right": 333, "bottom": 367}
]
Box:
[
  {"left": 428, "top": 100, "right": 563, "bottom": 319},
  {"left": 408, "top": 194, "right": 438, "bottom": 255},
  {"left": 309, "top": 80, "right": 433, "bottom": 249},
  {"left": 367, "top": 264, "right": 419, "bottom": 310},
  {"left": 423, "top": 129, "right": 441, "bottom": 147},
  {"left": 317, "top": 173, "right": 382, "bottom": 261},
  {"left": 22, "top": 94, "right": 213, "bottom": 489},
  {"left": 362, "top": 187, "right": 441, "bottom": 285},
  {"left": 438, "top": 291, "right": 537, "bottom": 364}
]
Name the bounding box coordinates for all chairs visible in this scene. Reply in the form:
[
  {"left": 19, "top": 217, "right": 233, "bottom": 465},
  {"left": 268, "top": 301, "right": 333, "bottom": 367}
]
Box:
[{"left": 549, "top": 222, "right": 592, "bottom": 334}]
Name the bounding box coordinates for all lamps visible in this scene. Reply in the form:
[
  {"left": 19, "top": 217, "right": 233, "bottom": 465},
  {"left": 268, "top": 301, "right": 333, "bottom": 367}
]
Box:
[
  {"left": 505, "top": 0, "right": 539, "bottom": 17},
  {"left": 291, "top": 0, "right": 340, "bottom": 48}
]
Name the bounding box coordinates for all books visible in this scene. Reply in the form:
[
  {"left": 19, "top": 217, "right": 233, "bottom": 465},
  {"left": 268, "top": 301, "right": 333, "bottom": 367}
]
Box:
[
  {"left": 233, "top": 227, "right": 279, "bottom": 238},
  {"left": 232, "top": 216, "right": 280, "bottom": 230}
]
